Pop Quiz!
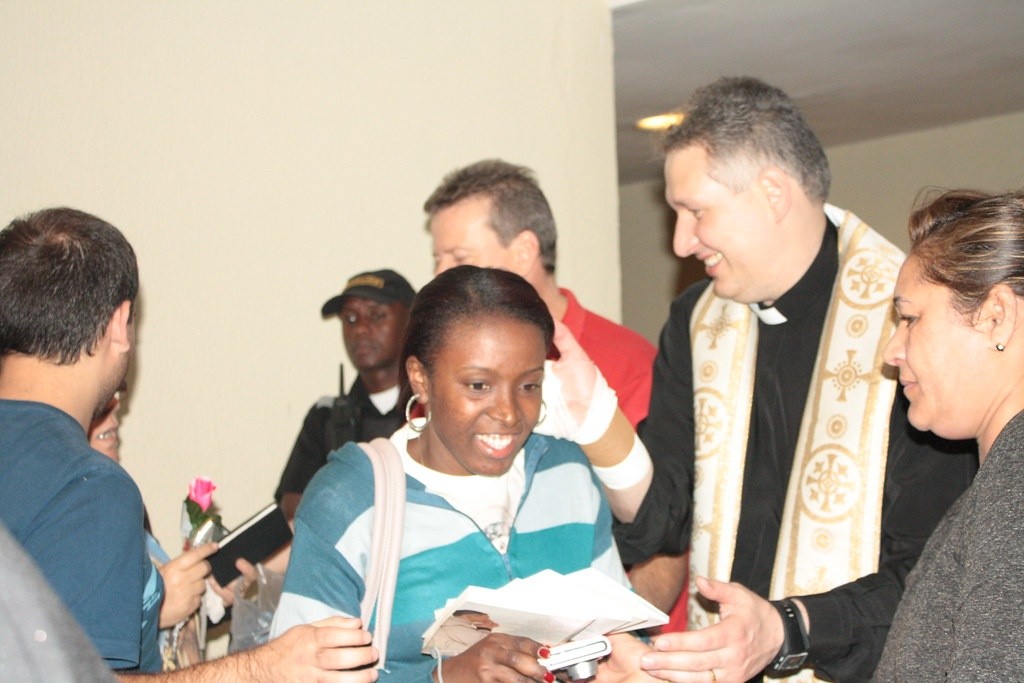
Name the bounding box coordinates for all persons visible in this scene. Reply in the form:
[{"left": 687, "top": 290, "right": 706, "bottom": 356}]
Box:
[
  {"left": 588, "top": 190, "right": 1024, "bottom": 683},
  {"left": 268, "top": 265, "right": 651, "bottom": 683},
  {"left": 552, "top": 76, "right": 981, "bottom": 683},
  {"left": 407, "top": 160, "right": 690, "bottom": 634},
  {"left": 0, "top": 206, "right": 380, "bottom": 683},
  {"left": 273, "top": 269, "right": 417, "bottom": 535}
]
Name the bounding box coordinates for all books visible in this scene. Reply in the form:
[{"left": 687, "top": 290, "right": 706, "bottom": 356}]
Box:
[
  {"left": 420, "top": 568, "right": 670, "bottom": 657},
  {"left": 208, "top": 503, "right": 293, "bottom": 586}
]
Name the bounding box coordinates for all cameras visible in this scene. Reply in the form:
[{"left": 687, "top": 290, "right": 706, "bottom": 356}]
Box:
[{"left": 537, "top": 636, "right": 612, "bottom": 680}]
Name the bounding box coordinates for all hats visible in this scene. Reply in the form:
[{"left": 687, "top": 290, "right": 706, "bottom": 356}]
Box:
[{"left": 320, "top": 269, "right": 416, "bottom": 316}]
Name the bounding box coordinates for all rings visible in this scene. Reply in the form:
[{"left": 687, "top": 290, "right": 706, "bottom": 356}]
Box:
[{"left": 710, "top": 670, "right": 716, "bottom": 683}]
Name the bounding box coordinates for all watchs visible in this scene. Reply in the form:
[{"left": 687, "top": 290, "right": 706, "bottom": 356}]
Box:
[{"left": 769, "top": 600, "right": 810, "bottom": 670}]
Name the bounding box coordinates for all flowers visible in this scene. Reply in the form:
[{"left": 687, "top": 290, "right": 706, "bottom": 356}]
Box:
[{"left": 185, "top": 477, "right": 221, "bottom": 532}]
